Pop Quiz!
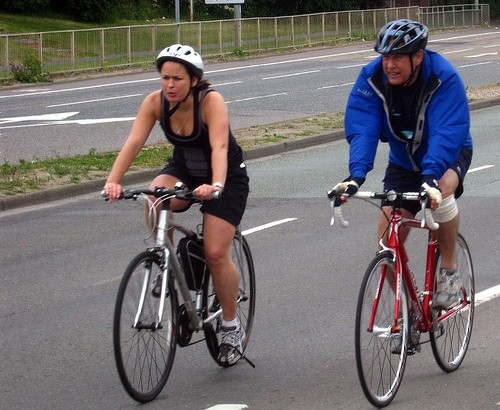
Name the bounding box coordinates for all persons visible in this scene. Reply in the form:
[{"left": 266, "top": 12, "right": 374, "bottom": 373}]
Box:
[
  {"left": 328, "top": 20, "right": 472, "bottom": 354},
  {"left": 103, "top": 44, "right": 250, "bottom": 365}
]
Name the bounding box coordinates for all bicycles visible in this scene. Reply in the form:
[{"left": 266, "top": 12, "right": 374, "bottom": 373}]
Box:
[
  {"left": 328, "top": 180, "right": 476, "bottom": 408},
  {"left": 101, "top": 182, "right": 257, "bottom": 405}
]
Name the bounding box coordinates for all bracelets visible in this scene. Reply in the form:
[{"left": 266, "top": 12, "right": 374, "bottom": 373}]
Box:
[{"left": 213, "top": 182, "right": 224, "bottom": 190}]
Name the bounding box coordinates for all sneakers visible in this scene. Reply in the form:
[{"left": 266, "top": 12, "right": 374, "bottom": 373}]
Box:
[
  {"left": 217, "top": 321, "right": 245, "bottom": 366},
  {"left": 390, "top": 332, "right": 417, "bottom": 353},
  {"left": 151, "top": 272, "right": 171, "bottom": 299},
  {"left": 432, "top": 268, "right": 460, "bottom": 307}
]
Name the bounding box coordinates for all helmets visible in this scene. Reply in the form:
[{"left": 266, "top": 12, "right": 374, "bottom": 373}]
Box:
[
  {"left": 374, "top": 17, "right": 429, "bottom": 55},
  {"left": 156, "top": 43, "right": 204, "bottom": 78}
]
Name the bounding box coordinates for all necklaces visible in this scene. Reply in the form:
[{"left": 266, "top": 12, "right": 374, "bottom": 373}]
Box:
[{"left": 172, "top": 113, "right": 189, "bottom": 136}]
